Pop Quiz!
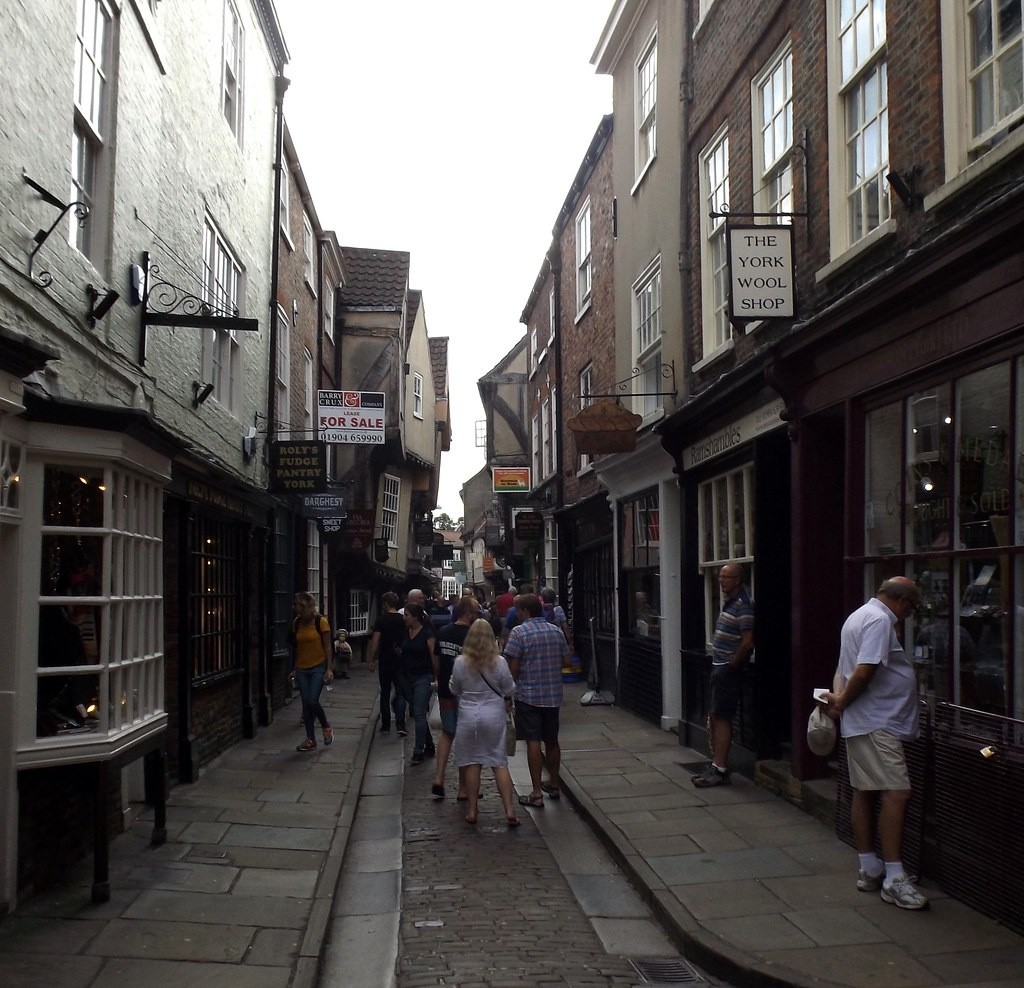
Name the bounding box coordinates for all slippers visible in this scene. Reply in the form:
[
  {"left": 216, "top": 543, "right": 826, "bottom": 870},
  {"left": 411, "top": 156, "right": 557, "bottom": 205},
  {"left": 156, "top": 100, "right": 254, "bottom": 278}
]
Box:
[
  {"left": 508, "top": 818, "right": 521, "bottom": 826},
  {"left": 464, "top": 816, "right": 476, "bottom": 824}
]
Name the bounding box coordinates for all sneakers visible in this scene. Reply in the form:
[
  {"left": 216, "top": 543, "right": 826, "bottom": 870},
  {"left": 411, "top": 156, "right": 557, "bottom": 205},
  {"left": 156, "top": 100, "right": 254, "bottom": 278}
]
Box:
[
  {"left": 322, "top": 727, "right": 334, "bottom": 746},
  {"left": 880, "top": 872, "right": 928, "bottom": 909},
  {"left": 855, "top": 869, "right": 887, "bottom": 892},
  {"left": 691, "top": 764, "right": 712, "bottom": 784},
  {"left": 295, "top": 738, "right": 317, "bottom": 750},
  {"left": 693, "top": 764, "right": 731, "bottom": 788}
]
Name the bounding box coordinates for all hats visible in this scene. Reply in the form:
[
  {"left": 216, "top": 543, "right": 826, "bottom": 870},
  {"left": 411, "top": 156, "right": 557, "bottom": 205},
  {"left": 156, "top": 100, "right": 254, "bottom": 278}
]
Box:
[{"left": 807, "top": 705, "right": 835, "bottom": 755}]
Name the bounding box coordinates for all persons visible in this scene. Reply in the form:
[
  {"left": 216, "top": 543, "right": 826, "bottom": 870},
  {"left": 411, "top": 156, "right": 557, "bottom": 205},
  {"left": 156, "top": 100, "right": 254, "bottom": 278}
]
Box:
[
  {"left": 501, "top": 594, "right": 573, "bottom": 808},
  {"left": 690, "top": 563, "right": 756, "bottom": 787},
  {"left": 289, "top": 591, "right": 335, "bottom": 751},
  {"left": 448, "top": 619, "right": 523, "bottom": 827},
  {"left": 819, "top": 576, "right": 928, "bottom": 910},
  {"left": 405, "top": 584, "right": 573, "bottom": 668},
  {"left": 333, "top": 628, "right": 353, "bottom": 679},
  {"left": 367, "top": 592, "right": 408, "bottom": 736},
  {"left": 431, "top": 596, "right": 484, "bottom": 802},
  {"left": 35, "top": 551, "right": 111, "bottom": 733},
  {"left": 910, "top": 576, "right": 1024, "bottom": 721},
  {"left": 393, "top": 604, "right": 441, "bottom": 764}
]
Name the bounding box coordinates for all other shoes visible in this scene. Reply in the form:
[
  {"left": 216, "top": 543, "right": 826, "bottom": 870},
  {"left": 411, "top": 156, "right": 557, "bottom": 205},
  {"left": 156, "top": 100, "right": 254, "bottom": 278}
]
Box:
[
  {"left": 396, "top": 725, "right": 407, "bottom": 736},
  {"left": 378, "top": 722, "right": 390, "bottom": 734},
  {"left": 424, "top": 746, "right": 435, "bottom": 757},
  {"left": 430, "top": 784, "right": 444, "bottom": 797},
  {"left": 456, "top": 793, "right": 483, "bottom": 801},
  {"left": 409, "top": 750, "right": 423, "bottom": 763}
]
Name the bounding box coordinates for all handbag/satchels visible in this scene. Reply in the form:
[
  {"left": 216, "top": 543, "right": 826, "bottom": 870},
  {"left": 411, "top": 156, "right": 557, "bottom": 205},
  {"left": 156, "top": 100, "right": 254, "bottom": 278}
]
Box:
[
  {"left": 429, "top": 697, "right": 442, "bottom": 729},
  {"left": 504, "top": 711, "right": 516, "bottom": 757}
]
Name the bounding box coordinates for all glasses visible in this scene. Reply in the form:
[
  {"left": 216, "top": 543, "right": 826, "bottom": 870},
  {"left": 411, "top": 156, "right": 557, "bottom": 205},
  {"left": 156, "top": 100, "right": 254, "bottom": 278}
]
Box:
[{"left": 717, "top": 574, "right": 736, "bottom": 581}]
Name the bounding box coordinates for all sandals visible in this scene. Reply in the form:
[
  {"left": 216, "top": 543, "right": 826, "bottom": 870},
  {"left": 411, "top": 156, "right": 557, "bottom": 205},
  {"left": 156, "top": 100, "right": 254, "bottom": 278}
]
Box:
[
  {"left": 540, "top": 780, "right": 559, "bottom": 799},
  {"left": 518, "top": 791, "right": 543, "bottom": 807}
]
